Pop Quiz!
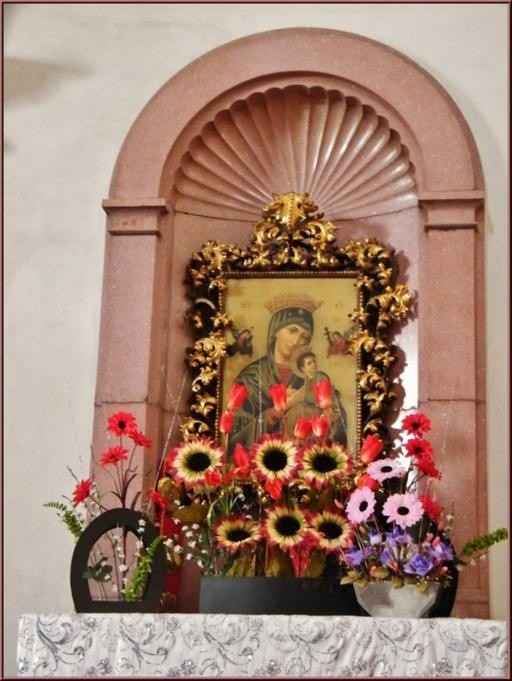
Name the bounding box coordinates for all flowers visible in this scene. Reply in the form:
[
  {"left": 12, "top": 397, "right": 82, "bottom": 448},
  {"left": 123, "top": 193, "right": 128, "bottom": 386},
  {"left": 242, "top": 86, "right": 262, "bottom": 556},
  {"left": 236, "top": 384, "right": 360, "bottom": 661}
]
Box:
[{"left": 43, "top": 385, "right": 509, "bottom": 606}]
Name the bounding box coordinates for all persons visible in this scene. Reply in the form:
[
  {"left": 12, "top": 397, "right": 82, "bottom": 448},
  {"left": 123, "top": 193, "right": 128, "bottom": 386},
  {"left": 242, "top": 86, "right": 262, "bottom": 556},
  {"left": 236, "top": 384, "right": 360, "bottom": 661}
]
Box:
[{"left": 225, "top": 309, "right": 346, "bottom": 452}]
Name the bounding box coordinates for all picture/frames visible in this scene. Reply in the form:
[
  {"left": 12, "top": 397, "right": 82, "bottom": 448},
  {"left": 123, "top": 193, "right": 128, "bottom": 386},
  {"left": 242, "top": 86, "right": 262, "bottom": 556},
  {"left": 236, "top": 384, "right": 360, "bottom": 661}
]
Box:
[{"left": 181, "top": 192, "right": 413, "bottom": 498}]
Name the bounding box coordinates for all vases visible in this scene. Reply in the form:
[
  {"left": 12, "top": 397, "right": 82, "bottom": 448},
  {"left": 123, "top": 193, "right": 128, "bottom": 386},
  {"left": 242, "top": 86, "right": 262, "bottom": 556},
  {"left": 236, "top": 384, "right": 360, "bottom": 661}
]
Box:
[{"left": 197, "top": 575, "right": 441, "bottom": 619}]
